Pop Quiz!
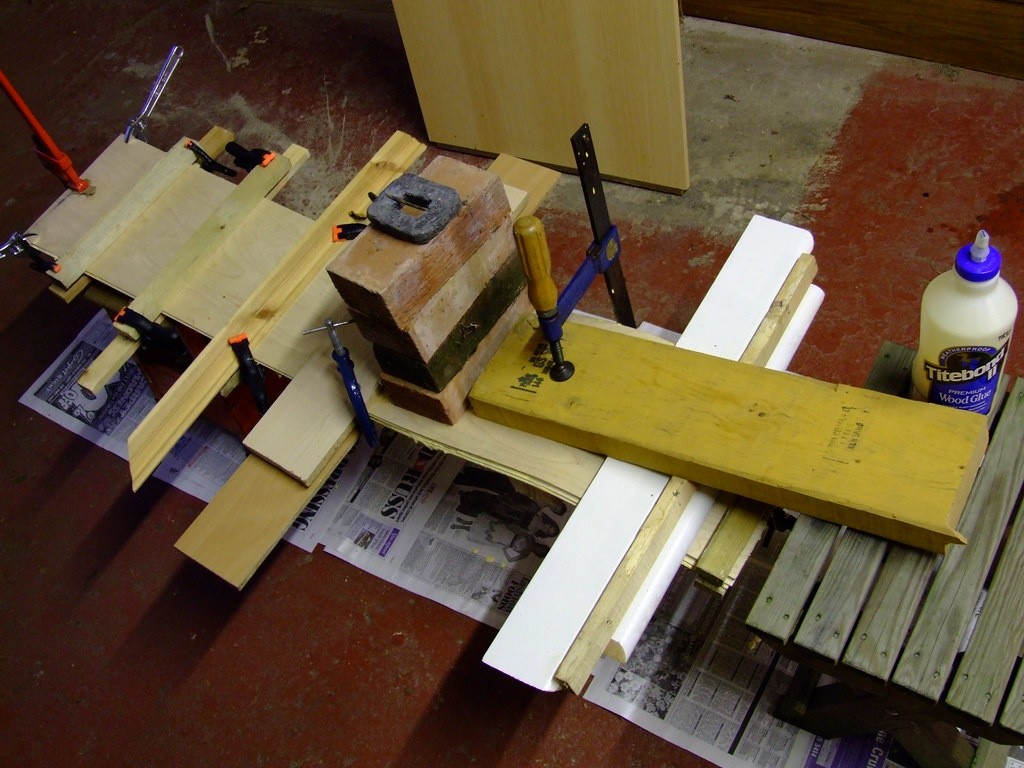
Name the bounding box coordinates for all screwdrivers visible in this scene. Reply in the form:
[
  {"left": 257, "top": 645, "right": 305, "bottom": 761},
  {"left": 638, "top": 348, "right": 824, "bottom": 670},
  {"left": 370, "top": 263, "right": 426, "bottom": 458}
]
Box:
[{"left": 512, "top": 215, "right": 567, "bottom": 371}]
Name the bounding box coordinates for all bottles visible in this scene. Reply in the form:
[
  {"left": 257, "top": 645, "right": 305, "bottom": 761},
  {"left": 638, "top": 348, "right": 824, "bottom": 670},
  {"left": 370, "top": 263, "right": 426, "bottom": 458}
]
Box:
[{"left": 910, "top": 230, "right": 1017, "bottom": 416}]
